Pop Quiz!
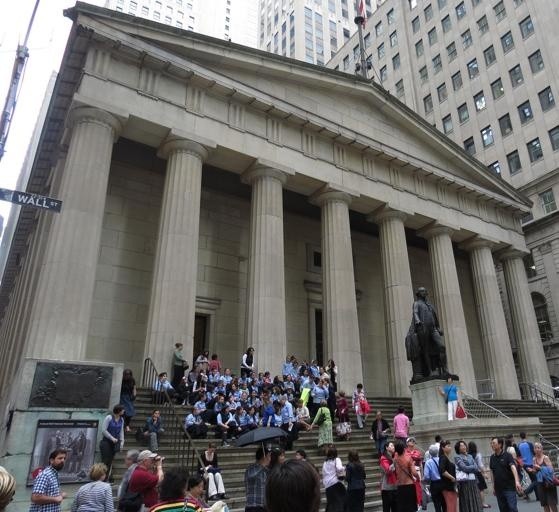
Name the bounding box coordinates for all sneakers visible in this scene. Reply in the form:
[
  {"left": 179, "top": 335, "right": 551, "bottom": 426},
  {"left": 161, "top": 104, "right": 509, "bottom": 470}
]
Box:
[
  {"left": 218, "top": 493, "right": 229, "bottom": 499},
  {"left": 209, "top": 494, "right": 220, "bottom": 500}
]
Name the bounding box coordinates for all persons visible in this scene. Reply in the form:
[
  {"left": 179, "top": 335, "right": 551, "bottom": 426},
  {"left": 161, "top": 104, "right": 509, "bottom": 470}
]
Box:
[
  {"left": 71, "top": 462, "right": 115, "bottom": 512},
  {"left": 0, "top": 466, "right": 16, "bottom": 512},
  {"left": 152, "top": 344, "right": 368, "bottom": 457},
  {"left": 29, "top": 449, "right": 67, "bottom": 512},
  {"left": 413, "top": 287, "right": 453, "bottom": 377},
  {"left": 244, "top": 448, "right": 366, "bottom": 512},
  {"left": 442, "top": 374, "right": 459, "bottom": 421},
  {"left": 369, "top": 406, "right": 559, "bottom": 512},
  {"left": 99, "top": 403, "right": 126, "bottom": 482},
  {"left": 116, "top": 368, "right": 230, "bottom": 512}
]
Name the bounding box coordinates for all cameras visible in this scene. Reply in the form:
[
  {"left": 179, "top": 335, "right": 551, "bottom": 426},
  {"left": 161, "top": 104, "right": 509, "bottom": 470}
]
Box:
[{"left": 153, "top": 456, "right": 166, "bottom": 462}]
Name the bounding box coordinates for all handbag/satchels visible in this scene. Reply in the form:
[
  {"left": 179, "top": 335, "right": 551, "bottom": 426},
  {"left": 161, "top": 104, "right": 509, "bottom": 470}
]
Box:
[
  {"left": 111, "top": 435, "right": 120, "bottom": 452},
  {"left": 335, "top": 413, "right": 352, "bottom": 436},
  {"left": 456, "top": 402, "right": 465, "bottom": 418},
  {"left": 512, "top": 458, "right": 534, "bottom": 497},
  {"left": 207, "top": 467, "right": 221, "bottom": 475},
  {"left": 119, "top": 492, "right": 143, "bottom": 512},
  {"left": 454, "top": 456, "right": 476, "bottom": 482},
  {"left": 446, "top": 398, "right": 448, "bottom": 404},
  {"left": 409, "top": 472, "right": 420, "bottom": 483},
  {"left": 317, "top": 407, "right": 326, "bottom": 426},
  {"left": 544, "top": 475, "right": 559, "bottom": 488},
  {"left": 335, "top": 457, "right": 347, "bottom": 480}
]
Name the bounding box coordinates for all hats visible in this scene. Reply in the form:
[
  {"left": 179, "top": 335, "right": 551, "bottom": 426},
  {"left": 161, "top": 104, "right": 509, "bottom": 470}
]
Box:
[{"left": 137, "top": 450, "right": 158, "bottom": 460}]
